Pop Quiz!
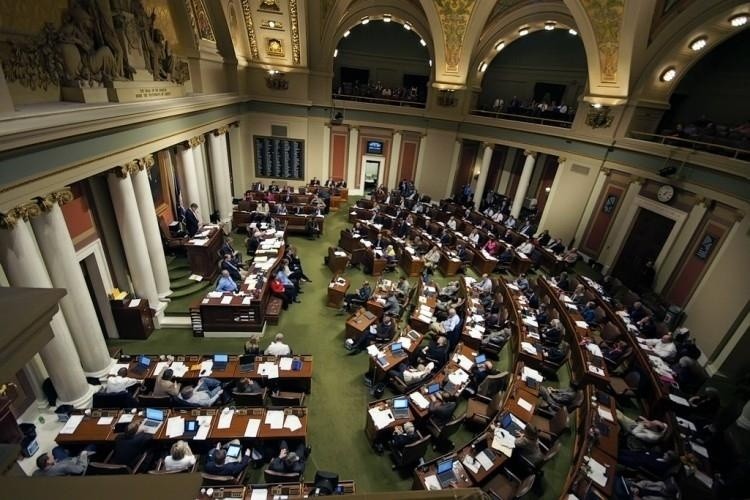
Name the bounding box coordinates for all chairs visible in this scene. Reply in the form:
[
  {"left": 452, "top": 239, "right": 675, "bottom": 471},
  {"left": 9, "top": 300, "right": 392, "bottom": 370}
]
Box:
[
  {"left": 199, "top": 225, "right": 288, "bottom": 332},
  {"left": 54, "top": 355, "right": 313, "bottom": 483},
  {"left": 158, "top": 207, "right": 189, "bottom": 258},
  {"left": 231, "top": 180, "right": 348, "bottom": 234},
  {"left": 338, "top": 188, "right": 733, "bottom": 500}
]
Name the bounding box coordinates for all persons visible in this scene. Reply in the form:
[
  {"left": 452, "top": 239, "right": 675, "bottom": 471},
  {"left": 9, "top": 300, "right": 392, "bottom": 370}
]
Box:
[
  {"left": 324, "top": 79, "right": 749, "bottom": 500},
  {"left": 184, "top": 177, "right": 324, "bottom": 304},
  {"left": 33, "top": 334, "right": 312, "bottom": 482}
]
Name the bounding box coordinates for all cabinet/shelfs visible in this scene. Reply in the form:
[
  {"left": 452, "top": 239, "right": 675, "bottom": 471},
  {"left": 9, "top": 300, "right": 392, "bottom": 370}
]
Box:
[{"left": 112, "top": 299, "right": 154, "bottom": 339}]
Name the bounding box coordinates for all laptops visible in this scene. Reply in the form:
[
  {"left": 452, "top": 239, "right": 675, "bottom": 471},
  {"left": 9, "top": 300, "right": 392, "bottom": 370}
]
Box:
[
  {"left": 130, "top": 353, "right": 255, "bottom": 437},
  {"left": 391, "top": 342, "right": 536, "bottom": 491}
]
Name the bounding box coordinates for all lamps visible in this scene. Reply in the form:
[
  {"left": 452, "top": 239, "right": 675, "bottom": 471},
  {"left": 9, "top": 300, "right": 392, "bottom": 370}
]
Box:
[
  {"left": 662, "top": 68, "right": 676, "bottom": 82},
  {"left": 691, "top": 39, "right": 706, "bottom": 52},
  {"left": 731, "top": 16, "right": 747, "bottom": 27}
]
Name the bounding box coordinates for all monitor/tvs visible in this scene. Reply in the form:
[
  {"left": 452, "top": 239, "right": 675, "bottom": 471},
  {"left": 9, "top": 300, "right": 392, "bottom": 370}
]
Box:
[{"left": 368, "top": 140, "right": 384, "bottom": 154}]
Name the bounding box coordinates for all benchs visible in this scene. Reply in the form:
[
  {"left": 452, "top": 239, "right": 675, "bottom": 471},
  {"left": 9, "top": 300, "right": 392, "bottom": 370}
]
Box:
[
  {"left": 236, "top": 222, "right": 321, "bottom": 238},
  {"left": 265, "top": 244, "right": 296, "bottom": 326}
]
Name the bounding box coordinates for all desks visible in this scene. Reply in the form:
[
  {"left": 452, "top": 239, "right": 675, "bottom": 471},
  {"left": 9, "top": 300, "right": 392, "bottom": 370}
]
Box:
[
  {"left": 184, "top": 222, "right": 225, "bottom": 276},
  {"left": 327, "top": 247, "right": 350, "bottom": 308}
]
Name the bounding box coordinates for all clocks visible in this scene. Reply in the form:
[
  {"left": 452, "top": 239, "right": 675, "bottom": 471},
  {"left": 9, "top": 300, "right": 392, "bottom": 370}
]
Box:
[{"left": 657, "top": 185, "right": 674, "bottom": 202}]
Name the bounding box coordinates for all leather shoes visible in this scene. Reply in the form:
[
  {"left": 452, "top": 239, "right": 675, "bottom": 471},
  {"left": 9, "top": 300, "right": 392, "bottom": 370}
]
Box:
[
  {"left": 222, "top": 381, "right": 232, "bottom": 388},
  {"left": 224, "top": 398, "right": 233, "bottom": 404}
]
